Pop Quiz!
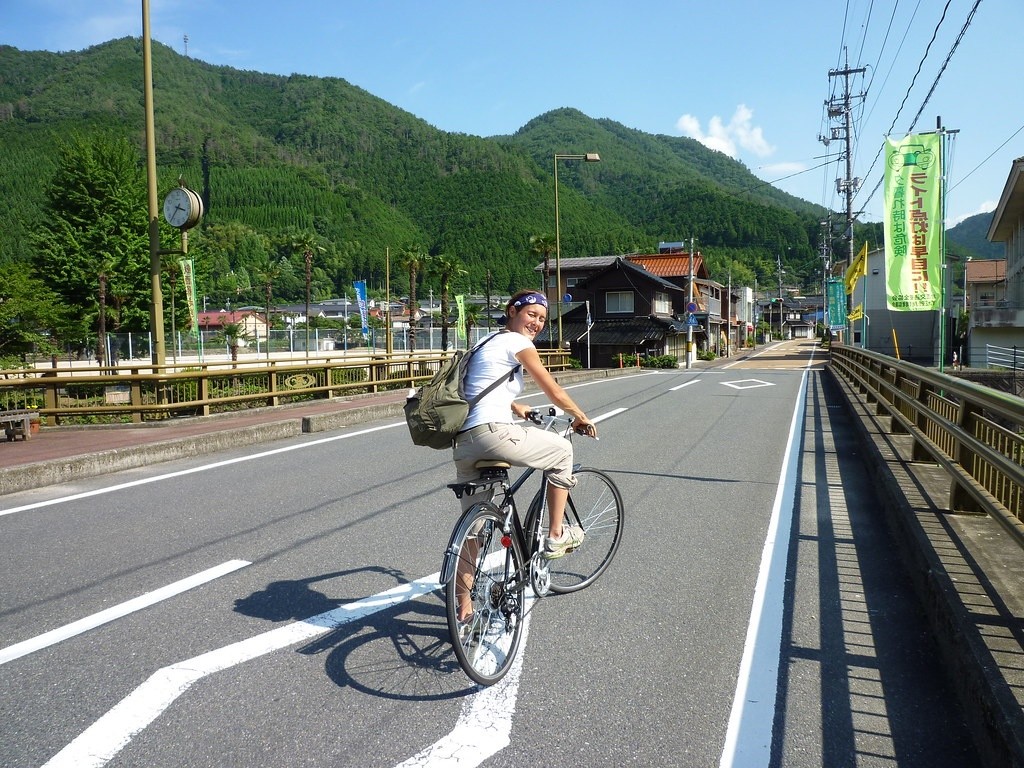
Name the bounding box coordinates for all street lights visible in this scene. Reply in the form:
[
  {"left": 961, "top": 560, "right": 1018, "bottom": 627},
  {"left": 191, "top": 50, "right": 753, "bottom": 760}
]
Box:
[{"left": 554, "top": 153, "right": 600, "bottom": 347}]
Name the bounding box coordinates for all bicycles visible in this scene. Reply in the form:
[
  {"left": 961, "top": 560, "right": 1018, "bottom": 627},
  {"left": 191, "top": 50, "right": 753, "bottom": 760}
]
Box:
[{"left": 438, "top": 405, "right": 626, "bottom": 687}]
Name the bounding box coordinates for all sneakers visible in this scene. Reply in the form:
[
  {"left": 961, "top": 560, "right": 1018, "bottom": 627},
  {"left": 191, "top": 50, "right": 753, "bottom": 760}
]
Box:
[
  {"left": 541, "top": 523, "right": 584, "bottom": 560},
  {"left": 456, "top": 610, "right": 488, "bottom": 644}
]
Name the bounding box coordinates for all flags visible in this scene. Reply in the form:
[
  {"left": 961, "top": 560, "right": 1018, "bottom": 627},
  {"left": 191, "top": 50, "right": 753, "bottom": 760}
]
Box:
[
  {"left": 178, "top": 259, "right": 198, "bottom": 340},
  {"left": 352, "top": 281, "right": 369, "bottom": 341},
  {"left": 826, "top": 281, "right": 848, "bottom": 331},
  {"left": 454, "top": 295, "right": 467, "bottom": 341},
  {"left": 880, "top": 132, "right": 947, "bottom": 316}
]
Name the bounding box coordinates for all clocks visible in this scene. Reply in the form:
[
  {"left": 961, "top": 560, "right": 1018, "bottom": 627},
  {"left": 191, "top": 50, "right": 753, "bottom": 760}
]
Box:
[{"left": 163, "top": 188, "right": 204, "bottom": 228}]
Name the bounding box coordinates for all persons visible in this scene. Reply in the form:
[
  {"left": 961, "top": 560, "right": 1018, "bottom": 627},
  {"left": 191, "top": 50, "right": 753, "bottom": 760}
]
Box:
[{"left": 444, "top": 289, "right": 598, "bottom": 641}]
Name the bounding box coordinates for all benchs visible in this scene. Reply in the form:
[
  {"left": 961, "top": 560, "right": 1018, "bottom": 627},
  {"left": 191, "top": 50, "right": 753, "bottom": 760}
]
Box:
[{"left": 0, "top": 412, "right": 39, "bottom": 440}]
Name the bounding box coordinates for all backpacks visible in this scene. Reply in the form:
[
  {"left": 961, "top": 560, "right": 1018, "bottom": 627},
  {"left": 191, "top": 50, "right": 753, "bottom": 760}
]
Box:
[{"left": 402, "top": 332, "right": 523, "bottom": 450}]
